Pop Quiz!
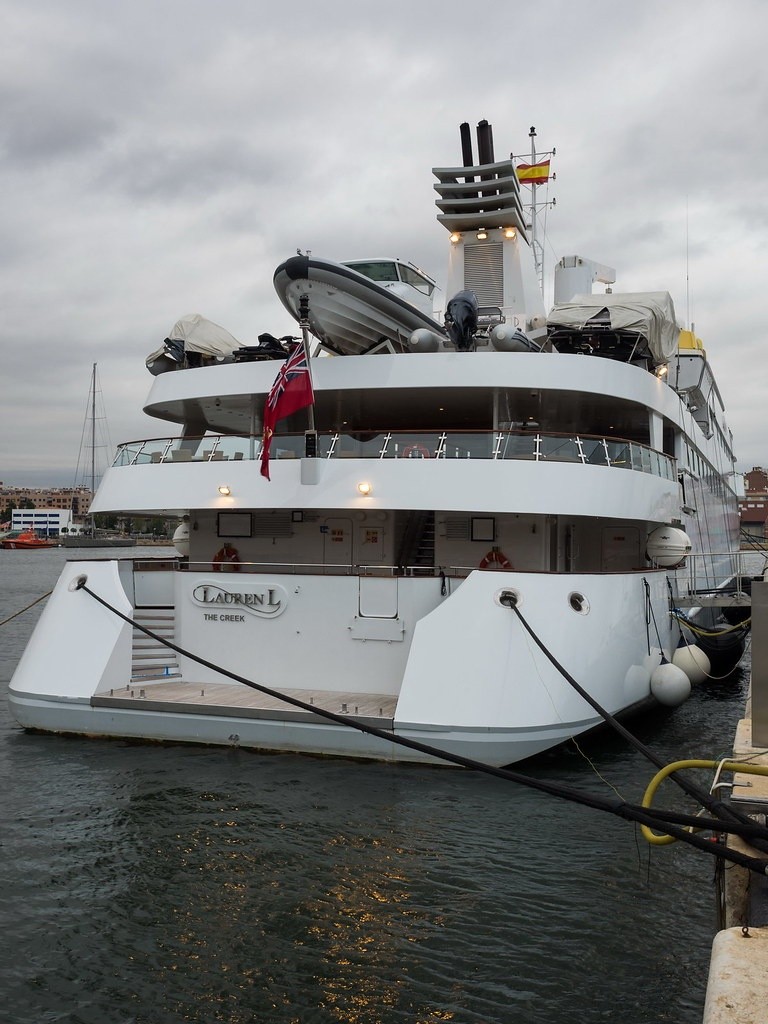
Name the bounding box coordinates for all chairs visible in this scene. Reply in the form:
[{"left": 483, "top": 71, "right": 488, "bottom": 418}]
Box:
[{"left": 150, "top": 448, "right": 243, "bottom": 463}]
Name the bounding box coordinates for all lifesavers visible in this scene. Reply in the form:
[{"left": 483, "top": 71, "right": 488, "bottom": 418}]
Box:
[
  {"left": 480, "top": 551, "right": 511, "bottom": 569},
  {"left": 212, "top": 545, "right": 242, "bottom": 574},
  {"left": 403, "top": 444, "right": 431, "bottom": 459}
]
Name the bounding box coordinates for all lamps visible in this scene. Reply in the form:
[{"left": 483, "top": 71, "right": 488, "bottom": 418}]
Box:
[
  {"left": 688, "top": 405, "right": 698, "bottom": 412},
  {"left": 476, "top": 231, "right": 488, "bottom": 240}
]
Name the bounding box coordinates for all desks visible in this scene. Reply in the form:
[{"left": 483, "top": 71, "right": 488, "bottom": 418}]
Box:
[{"left": 163, "top": 455, "right": 229, "bottom": 461}]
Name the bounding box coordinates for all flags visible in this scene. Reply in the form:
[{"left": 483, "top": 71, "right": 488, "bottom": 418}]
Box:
[
  {"left": 516, "top": 159, "right": 549, "bottom": 184},
  {"left": 260, "top": 338, "right": 315, "bottom": 481}
]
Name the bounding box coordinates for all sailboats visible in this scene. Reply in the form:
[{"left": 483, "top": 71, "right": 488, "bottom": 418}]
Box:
[{"left": 62, "top": 363, "right": 137, "bottom": 547}]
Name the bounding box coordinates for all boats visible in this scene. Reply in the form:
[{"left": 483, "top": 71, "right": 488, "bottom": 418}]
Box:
[
  {"left": 2, "top": 530, "right": 59, "bottom": 549},
  {"left": 8, "top": 118, "right": 741, "bottom": 770}
]
